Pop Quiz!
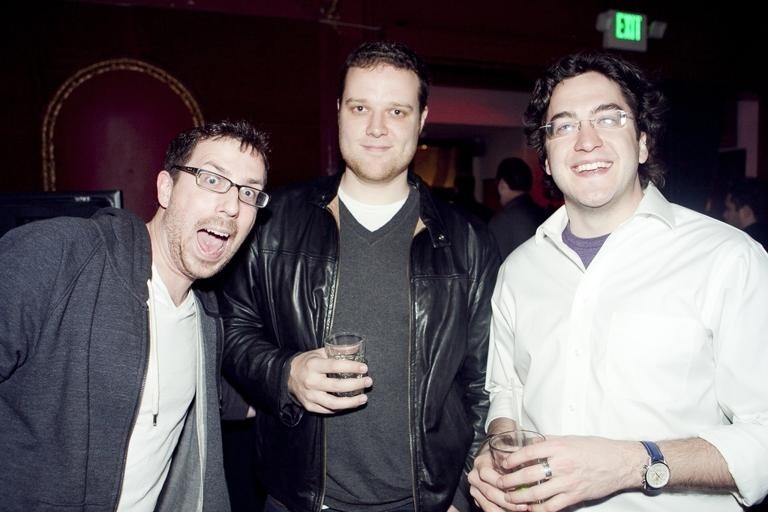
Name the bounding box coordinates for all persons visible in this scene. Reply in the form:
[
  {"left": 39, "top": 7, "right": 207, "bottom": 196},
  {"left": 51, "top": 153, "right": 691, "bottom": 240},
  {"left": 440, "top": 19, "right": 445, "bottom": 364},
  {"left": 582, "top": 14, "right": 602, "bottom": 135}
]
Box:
[
  {"left": 0, "top": 114, "right": 274, "bottom": 512},
  {"left": 466, "top": 47, "right": 767, "bottom": 511},
  {"left": 476, "top": 154, "right": 767, "bottom": 260},
  {"left": 212, "top": 37, "right": 502, "bottom": 512}
]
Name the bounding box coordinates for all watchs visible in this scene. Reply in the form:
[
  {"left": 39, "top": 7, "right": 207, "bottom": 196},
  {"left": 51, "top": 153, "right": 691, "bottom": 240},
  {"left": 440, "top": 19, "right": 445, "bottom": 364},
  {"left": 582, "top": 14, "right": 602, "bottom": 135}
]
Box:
[{"left": 639, "top": 438, "right": 671, "bottom": 497}]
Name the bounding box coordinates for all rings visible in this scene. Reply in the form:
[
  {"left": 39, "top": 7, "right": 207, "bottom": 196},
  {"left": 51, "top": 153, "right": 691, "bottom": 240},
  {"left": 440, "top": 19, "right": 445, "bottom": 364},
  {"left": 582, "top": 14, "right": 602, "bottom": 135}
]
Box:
[{"left": 543, "top": 461, "right": 551, "bottom": 477}]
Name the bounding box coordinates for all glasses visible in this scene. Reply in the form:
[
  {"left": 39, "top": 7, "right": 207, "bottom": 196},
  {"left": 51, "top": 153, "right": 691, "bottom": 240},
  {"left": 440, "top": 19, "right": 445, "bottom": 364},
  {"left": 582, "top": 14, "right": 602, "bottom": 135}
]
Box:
[
  {"left": 170, "top": 164, "right": 272, "bottom": 209},
  {"left": 538, "top": 109, "right": 634, "bottom": 140}
]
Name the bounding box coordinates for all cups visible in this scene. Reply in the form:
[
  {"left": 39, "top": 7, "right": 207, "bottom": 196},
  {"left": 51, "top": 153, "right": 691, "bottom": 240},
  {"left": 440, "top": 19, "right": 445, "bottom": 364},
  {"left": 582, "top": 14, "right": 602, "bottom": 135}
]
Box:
[
  {"left": 323, "top": 330, "right": 367, "bottom": 400},
  {"left": 486, "top": 429, "right": 549, "bottom": 506}
]
